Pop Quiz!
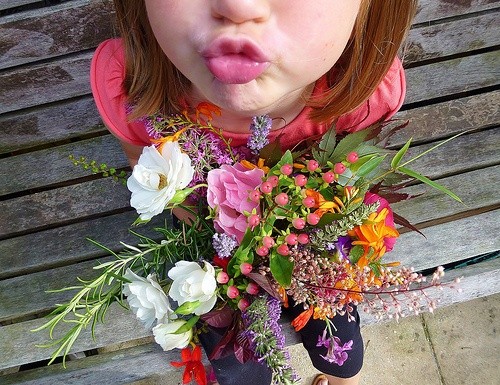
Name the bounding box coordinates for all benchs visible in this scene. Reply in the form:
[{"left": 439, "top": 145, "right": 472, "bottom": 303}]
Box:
[{"left": 0, "top": 0, "right": 500, "bottom": 385}]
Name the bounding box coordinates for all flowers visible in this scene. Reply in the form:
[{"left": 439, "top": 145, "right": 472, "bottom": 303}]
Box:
[{"left": 30, "top": 98, "right": 469, "bottom": 385}]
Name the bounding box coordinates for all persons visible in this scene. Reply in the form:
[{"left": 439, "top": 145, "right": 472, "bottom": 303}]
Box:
[{"left": 90, "top": 0, "right": 420, "bottom": 385}]
